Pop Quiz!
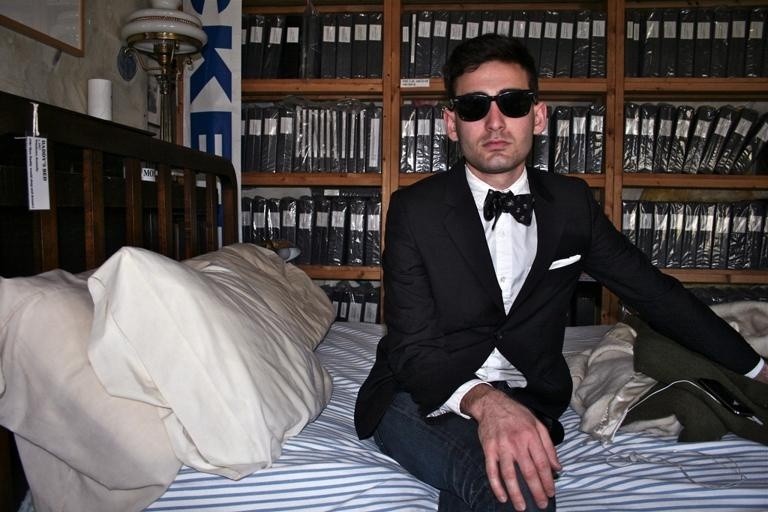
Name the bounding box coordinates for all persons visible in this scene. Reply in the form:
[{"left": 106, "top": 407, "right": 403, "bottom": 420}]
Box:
[{"left": 356, "top": 34, "right": 768, "bottom": 512}]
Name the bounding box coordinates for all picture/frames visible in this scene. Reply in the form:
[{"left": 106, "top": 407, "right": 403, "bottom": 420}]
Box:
[{"left": 0, "top": 0, "right": 85, "bottom": 58}]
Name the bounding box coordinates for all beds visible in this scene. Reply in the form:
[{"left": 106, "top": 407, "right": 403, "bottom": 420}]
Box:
[{"left": 1, "top": 91, "right": 768, "bottom": 512}]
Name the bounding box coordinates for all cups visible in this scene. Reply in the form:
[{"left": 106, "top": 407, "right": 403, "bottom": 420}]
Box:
[{"left": 87, "top": 77, "right": 114, "bottom": 121}]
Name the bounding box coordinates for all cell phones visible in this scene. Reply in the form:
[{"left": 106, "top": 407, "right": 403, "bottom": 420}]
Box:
[{"left": 699, "top": 376, "right": 756, "bottom": 415}]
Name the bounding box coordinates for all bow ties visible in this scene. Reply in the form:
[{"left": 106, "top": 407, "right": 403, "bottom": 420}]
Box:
[{"left": 483, "top": 189, "right": 533, "bottom": 230}]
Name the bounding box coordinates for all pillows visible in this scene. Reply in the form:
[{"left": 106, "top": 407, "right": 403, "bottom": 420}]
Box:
[
  {"left": 0, "top": 269, "right": 189, "bottom": 512},
  {"left": 87, "top": 234, "right": 333, "bottom": 482}
]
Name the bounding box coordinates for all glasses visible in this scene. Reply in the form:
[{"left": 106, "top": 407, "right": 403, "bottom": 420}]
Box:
[{"left": 452, "top": 89, "right": 539, "bottom": 122}]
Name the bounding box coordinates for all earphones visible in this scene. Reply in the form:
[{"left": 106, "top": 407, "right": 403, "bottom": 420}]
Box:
[
  {"left": 672, "top": 449, "right": 677, "bottom": 452},
  {"left": 629, "top": 454, "right": 637, "bottom": 462}
]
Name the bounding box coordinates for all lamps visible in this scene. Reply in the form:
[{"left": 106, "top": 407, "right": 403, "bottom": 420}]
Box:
[
  {"left": 122, "top": 0, "right": 211, "bottom": 145},
  {"left": 250, "top": 193, "right": 302, "bottom": 263}
]
